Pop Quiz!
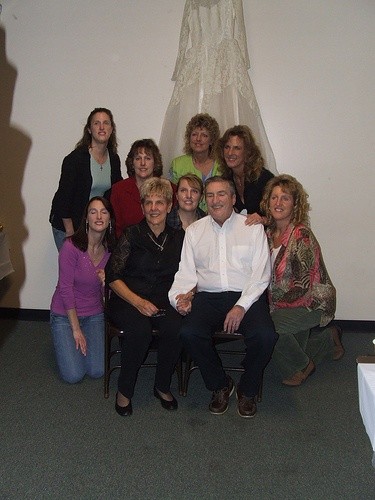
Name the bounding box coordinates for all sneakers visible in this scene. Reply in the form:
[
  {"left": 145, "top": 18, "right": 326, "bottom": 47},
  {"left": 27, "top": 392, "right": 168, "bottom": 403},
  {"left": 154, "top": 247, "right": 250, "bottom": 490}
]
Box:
[
  {"left": 235, "top": 385, "right": 258, "bottom": 419},
  {"left": 208, "top": 375, "right": 234, "bottom": 415}
]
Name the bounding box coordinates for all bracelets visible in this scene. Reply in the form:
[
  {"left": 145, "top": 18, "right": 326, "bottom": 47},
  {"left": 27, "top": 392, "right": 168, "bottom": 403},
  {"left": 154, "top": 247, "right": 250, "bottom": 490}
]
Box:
[{"left": 188, "top": 291, "right": 194, "bottom": 299}]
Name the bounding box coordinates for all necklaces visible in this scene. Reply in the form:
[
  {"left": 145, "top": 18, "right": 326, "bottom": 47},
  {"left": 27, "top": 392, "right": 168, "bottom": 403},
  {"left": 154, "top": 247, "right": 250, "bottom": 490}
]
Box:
[
  {"left": 100, "top": 164, "right": 104, "bottom": 172},
  {"left": 148, "top": 233, "right": 168, "bottom": 252}
]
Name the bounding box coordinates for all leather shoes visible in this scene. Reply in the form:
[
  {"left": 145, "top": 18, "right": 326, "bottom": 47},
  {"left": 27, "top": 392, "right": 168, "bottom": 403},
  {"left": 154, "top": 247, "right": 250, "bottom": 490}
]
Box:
[
  {"left": 282, "top": 360, "right": 318, "bottom": 386},
  {"left": 330, "top": 324, "right": 343, "bottom": 361},
  {"left": 156, "top": 385, "right": 179, "bottom": 411},
  {"left": 115, "top": 387, "right": 133, "bottom": 415}
]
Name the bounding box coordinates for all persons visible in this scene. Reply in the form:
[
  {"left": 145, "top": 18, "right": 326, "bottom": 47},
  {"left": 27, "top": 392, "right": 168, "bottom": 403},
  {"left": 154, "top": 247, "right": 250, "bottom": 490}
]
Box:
[
  {"left": 48, "top": 108, "right": 121, "bottom": 253},
  {"left": 260, "top": 174, "right": 345, "bottom": 386},
  {"left": 174, "top": 172, "right": 207, "bottom": 232},
  {"left": 103, "top": 177, "right": 184, "bottom": 417},
  {"left": 108, "top": 139, "right": 177, "bottom": 238},
  {"left": 167, "top": 176, "right": 279, "bottom": 418},
  {"left": 169, "top": 113, "right": 222, "bottom": 214},
  {"left": 214, "top": 125, "right": 276, "bottom": 231},
  {"left": 49, "top": 196, "right": 116, "bottom": 384}
]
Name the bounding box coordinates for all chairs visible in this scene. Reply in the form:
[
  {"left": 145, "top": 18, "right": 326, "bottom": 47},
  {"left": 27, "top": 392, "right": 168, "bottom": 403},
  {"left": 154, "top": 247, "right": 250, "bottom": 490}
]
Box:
[
  {"left": 183, "top": 328, "right": 262, "bottom": 403},
  {"left": 104, "top": 283, "right": 183, "bottom": 398}
]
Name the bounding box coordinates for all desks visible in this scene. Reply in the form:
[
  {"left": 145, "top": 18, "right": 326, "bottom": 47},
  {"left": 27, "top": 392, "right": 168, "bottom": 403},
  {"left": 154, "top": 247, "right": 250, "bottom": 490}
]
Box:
[{"left": 357, "top": 363, "right": 375, "bottom": 451}]
